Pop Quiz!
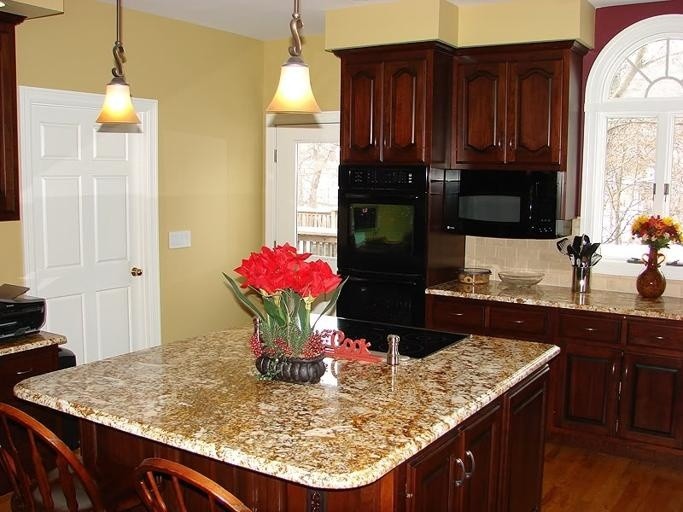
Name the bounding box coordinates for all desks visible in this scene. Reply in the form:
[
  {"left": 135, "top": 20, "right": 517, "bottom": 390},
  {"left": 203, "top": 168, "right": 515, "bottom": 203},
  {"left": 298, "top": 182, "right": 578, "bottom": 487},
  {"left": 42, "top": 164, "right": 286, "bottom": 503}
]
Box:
[{"left": 15, "top": 328, "right": 559, "bottom": 510}]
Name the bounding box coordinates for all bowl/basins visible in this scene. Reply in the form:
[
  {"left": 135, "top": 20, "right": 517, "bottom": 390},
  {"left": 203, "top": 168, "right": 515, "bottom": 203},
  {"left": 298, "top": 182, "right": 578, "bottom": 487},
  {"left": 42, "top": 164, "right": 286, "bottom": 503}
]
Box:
[
  {"left": 457, "top": 269, "right": 491, "bottom": 284},
  {"left": 496, "top": 287, "right": 547, "bottom": 300},
  {"left": 497, "top": 269, "right": 545, "bottom": 286}
]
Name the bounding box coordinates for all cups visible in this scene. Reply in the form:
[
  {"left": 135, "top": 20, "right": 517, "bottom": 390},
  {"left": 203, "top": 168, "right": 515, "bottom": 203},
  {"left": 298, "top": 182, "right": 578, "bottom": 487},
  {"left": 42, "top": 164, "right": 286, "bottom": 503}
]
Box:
[
  {"left": 572, "top": 266, "right": 591, "bottom": 293},
  {"left": 572, "top": 293, "right": 590, "bottom": 306}
]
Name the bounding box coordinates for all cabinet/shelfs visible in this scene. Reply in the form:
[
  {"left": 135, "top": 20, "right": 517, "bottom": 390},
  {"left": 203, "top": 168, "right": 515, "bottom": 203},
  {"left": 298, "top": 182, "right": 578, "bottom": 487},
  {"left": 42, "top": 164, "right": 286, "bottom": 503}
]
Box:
[
  {"left": 323, "top": 42, "right": 448, "bottom": 166},
  {"left": 0, "top": 11, "right": 30, "bottom": 222},
  {"left": 425, "top": 293, "right": 556, "bottom": 466},
  {"left": 558, "top": 304, "right": 681, "bottom": 466},
  {"left": 0, "top": 344, "right": 63, "bottom": 494},
  {"left": 453, "top": 42, "right": 591, "bottom": 173}
]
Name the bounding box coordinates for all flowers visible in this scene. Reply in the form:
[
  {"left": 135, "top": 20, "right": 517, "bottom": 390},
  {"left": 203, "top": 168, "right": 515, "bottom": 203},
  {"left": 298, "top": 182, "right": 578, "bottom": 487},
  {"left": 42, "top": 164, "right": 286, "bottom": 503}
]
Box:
[
  {"left": 629, "top": 216, "right": 683, "bottom": 265},
  {"left": 218, "top": 239, "right": 351, "bottom": 358}
]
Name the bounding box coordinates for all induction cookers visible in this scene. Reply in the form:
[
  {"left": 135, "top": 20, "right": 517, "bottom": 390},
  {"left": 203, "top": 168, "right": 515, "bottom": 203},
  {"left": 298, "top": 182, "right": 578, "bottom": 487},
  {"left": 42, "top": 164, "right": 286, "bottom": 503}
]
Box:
[{"left": 305, "top": 316, "right": 468, "bottom": 360}]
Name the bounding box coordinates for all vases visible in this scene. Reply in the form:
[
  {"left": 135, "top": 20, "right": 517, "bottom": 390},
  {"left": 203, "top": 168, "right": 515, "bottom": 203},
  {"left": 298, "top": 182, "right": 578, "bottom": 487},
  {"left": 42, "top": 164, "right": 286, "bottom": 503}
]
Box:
[
  {"left": 636, "top": 251, "right": 667, "bottom": 297},
  {"left": 253, "top": 351, "right": 328, "bottom": 384}
]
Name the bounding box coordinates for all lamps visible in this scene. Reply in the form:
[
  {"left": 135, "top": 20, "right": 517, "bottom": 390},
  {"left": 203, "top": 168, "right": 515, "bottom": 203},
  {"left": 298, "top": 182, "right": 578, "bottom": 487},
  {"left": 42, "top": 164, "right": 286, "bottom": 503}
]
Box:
[
  {"left": 97, "top": 1, "right": 145, "bottom": 125},
  {"left": 263, "top": 1, "right": 324, "bottom": 117}
]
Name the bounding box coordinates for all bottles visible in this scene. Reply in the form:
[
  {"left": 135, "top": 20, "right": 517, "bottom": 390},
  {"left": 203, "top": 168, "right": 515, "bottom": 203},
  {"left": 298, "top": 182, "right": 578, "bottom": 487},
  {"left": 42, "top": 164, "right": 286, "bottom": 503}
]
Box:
[{"left": 384, "top": 333, "right": 402, "bottom": 366}]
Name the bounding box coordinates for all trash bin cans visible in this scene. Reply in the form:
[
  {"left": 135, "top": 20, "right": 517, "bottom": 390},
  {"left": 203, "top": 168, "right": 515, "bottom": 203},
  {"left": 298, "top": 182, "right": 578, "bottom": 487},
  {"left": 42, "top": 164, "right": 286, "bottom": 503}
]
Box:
[{"left": 57, "top": 347, "right": 82, "bottom": 452}]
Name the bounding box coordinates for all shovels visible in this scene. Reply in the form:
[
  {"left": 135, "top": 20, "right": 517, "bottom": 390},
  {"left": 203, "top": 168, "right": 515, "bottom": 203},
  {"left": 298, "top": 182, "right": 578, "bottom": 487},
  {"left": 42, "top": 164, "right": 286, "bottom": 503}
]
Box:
[{"left": 556, "top": 234, "right": 602, "bottom": 268}]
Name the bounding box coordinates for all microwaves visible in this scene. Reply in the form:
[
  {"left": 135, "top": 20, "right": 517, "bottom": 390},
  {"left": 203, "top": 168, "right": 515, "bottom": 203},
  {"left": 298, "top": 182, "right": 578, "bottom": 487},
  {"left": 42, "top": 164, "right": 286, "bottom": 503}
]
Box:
[{"left": 435, "top": 169, "right": 572, "bottom": 235}]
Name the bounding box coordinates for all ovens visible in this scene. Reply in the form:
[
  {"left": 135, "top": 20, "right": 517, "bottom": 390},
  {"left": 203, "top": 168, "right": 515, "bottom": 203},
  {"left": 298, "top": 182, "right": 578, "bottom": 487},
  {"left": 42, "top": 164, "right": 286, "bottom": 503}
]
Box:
[{"left": 336, "top": 187, "right": 422, "bottom": 327}]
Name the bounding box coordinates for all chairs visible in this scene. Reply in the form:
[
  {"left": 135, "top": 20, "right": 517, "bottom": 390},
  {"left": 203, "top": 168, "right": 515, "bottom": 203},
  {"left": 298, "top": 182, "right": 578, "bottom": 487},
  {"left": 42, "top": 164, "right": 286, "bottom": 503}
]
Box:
[
  {"left": 0, "top": 402, "right": 111, "bottom": 512},
  {"left": 133, "top": 453, "right": 255, "bottom": 509}
]
